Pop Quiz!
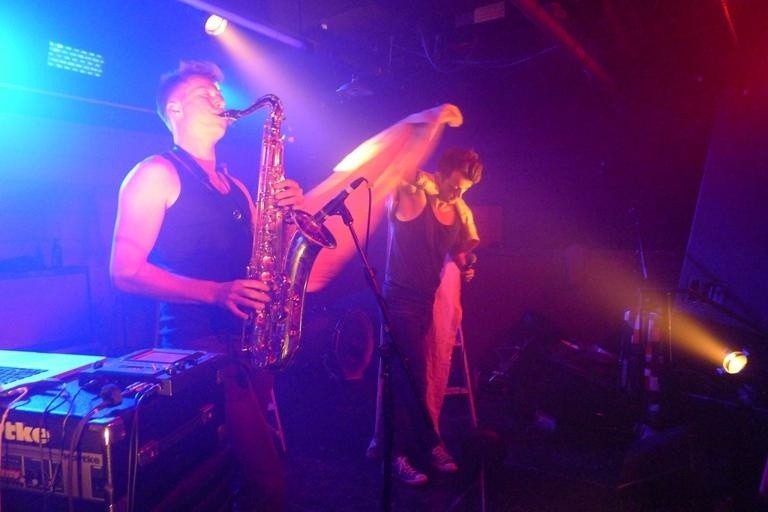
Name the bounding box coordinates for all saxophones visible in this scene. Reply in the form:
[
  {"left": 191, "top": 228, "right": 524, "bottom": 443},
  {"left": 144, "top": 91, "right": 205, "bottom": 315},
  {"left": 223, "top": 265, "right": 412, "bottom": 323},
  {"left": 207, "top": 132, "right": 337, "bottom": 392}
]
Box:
[{"left": 218, "top": 92, "right": 337, "bottom": 375}]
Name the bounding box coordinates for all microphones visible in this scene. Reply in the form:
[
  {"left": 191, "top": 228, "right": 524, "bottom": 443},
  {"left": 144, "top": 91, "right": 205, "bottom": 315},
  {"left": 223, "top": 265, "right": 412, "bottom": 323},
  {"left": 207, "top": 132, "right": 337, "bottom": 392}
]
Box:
[{"left": 304, "top": 176, "right": 366, "bottom": 239}]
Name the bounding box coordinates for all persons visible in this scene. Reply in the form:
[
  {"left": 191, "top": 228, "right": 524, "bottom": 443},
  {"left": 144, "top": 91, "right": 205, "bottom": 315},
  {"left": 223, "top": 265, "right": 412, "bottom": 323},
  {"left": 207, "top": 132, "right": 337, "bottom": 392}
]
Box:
[
  {"left": 376, "top": 146, "right": 486, "bottom": 490},
  {"left": 108, "top": 57, "right": 312, "bottom": 512}
]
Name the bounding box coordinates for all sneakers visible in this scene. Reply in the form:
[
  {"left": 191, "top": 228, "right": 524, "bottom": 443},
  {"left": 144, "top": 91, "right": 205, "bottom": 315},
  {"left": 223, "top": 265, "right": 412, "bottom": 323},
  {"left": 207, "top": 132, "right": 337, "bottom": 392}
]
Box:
[
  {"left": 428, "top": 442, "right": 459, "bottom": 473},
  {"left": 381, "top": 451, "right": 429, "bottom": 487}
]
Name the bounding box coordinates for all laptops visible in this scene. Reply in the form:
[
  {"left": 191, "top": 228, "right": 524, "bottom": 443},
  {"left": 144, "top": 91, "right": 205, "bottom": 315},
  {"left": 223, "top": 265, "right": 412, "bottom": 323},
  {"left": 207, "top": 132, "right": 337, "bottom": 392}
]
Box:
[{"left": 0, "top": 347, "right": 106, "bottom": 398}]
